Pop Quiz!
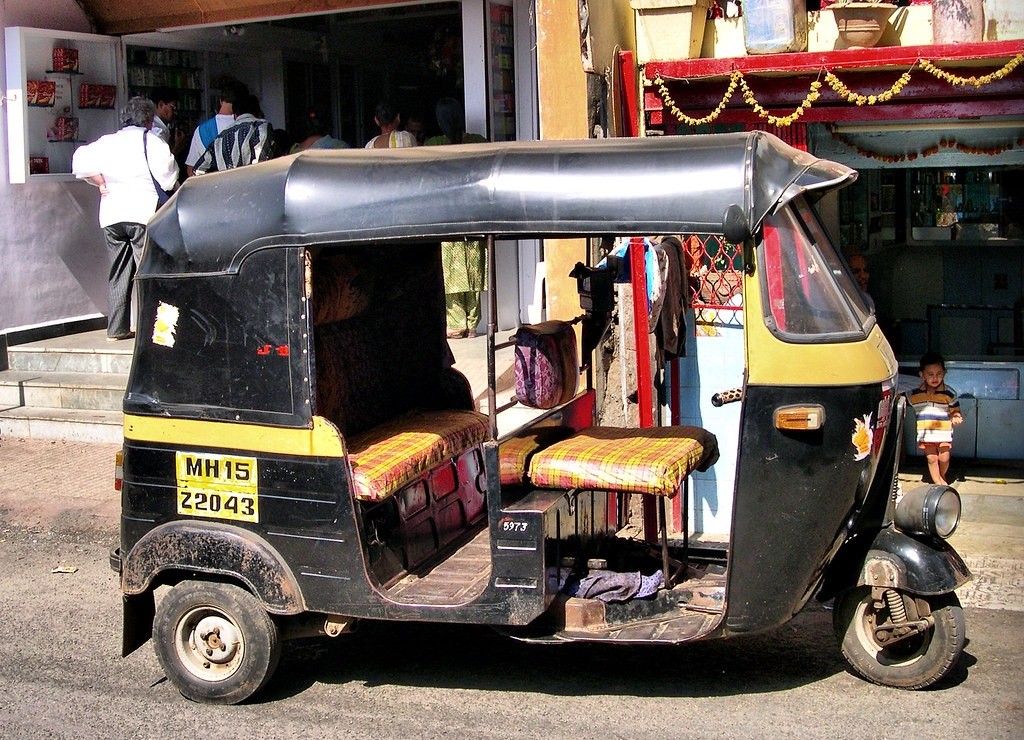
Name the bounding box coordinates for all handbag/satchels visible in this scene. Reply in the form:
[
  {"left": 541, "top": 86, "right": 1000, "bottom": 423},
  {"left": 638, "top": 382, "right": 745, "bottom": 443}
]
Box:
[{"left": 153, "top": 177, "right": 169, "bottom": 212}]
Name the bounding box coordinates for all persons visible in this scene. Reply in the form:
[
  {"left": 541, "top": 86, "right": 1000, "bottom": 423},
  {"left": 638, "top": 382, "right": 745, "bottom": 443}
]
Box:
[
  {"left": 844, "top": 254, "right": 868, "bottom": 294},
  {"left": 898, "top": 353, "right": 963, "bottom": 486},
  {"left": 71, "top": 96, "right": 180, "bottom": 339},
  {"left": 290, "top": 104, "right": 349, "bottom": 154},
  {"left": 402, "top": 113, "right": 429, "bottom": 147},
  {"left": 422, "top": 97, "right": 491, "bottom": 341},
  {"left": 150, "top": 91, "right": 188, "bottom": 156},
  {"left": 363, "top": 101, "right": 421, "bottom": 148},
  {"left": 184, "top": 81, "right": 291, "bottom": 176}
]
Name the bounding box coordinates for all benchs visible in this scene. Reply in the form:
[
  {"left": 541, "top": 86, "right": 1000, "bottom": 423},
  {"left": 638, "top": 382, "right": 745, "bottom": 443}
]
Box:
[{"left": 315, "top": 324, "right": 491, "bottom": 513}]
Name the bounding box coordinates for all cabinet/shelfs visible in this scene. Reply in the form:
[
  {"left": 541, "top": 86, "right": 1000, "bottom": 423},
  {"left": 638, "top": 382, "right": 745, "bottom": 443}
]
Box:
[
  {"left": 819, "top": 168, "right": 880, "bottom": 262},
  {"left": 905, "top": 168, "right": 1024, "bottom": 247},
  {"left": 4, "top": 26, "right": 244, "bottom": 189}
]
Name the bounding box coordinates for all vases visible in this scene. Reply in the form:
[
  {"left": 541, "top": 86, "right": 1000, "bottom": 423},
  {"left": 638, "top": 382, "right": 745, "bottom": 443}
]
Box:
[{"left": 825, "top": 1, "right": 898, "bottom": 49}]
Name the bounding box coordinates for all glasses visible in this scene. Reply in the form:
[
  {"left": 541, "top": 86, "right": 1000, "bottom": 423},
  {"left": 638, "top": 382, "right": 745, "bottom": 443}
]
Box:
[{"left": 168, "top": 103, "right": 177, "bottom": 111}]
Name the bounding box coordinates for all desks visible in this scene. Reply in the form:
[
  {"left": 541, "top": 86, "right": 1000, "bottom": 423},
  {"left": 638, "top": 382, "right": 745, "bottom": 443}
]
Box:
[
  {"left": 990, "top": 308, "right": 1024, "bottom": 357},
  {"left": 927, "top": 304, "right": 990, "bottom": 357}
]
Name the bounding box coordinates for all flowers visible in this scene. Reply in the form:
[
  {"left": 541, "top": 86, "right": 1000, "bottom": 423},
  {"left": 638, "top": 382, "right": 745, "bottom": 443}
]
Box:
[{"left": 819, "top": 0, "right": 897, "bottom": 11}]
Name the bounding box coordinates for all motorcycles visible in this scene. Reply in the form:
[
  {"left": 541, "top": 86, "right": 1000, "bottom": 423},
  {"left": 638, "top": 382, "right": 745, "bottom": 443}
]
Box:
[{"left": 111, "top": 130, "right": 973, "bottom": 706}]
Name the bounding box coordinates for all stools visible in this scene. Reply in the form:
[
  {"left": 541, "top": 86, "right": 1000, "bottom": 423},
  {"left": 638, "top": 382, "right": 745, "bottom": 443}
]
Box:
[
  {"left": 528, "top": 426, "right": 720, "bottom": 592},
  {"left": 497, "top": 436, "right": 542, "bottom": 487}
]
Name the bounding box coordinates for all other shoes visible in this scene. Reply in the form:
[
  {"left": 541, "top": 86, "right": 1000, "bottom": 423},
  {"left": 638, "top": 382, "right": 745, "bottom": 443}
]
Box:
[{"left": 106, "top": 331, "right": 136, "bottom": 342}]
